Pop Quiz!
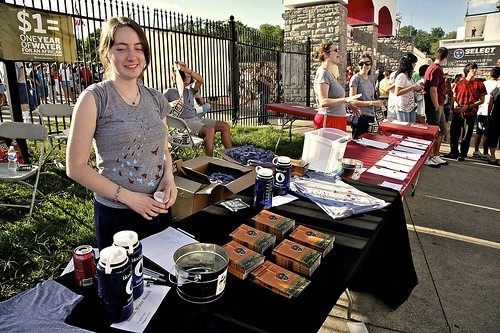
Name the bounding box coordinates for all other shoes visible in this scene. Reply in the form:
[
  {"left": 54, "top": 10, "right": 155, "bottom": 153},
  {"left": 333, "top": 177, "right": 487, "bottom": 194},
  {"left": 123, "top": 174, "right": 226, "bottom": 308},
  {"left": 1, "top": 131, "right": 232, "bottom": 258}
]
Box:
[
  {"left": 444, "top": 150, "right": 459, "bottom": 158},
  {"left": 458, "top": 153, "right": 467, "bottom": 158},
  {"left": 481, "top": 154, "right": 490, "bottom": 160},
  {"left": 473, "top": 151, "right": 482, "bottom": 157},
  {"left": 489, "top": 160, "right": 500, "bottom": 165}
]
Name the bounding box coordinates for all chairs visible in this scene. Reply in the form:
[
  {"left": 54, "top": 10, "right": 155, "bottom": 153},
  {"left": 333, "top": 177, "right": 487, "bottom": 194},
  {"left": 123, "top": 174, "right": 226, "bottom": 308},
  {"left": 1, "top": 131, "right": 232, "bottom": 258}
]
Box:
[
  {"left": 38, "top": 103, "right": 72, "bottom": 170},
  {"left": 166, "top": 116, "right": 205, "bottom": 160},
  {"left": 0, "top": 123, "right": 47, "bottom": 216}
]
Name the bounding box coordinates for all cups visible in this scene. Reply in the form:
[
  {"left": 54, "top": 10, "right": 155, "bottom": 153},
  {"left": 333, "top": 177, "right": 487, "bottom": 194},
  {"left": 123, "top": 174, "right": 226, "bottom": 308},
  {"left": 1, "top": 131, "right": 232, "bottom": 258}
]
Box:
[
  {"left": 368, "top": 123, "right": 379, "bottom": 135},
  {"left": 182, "top": 135, "right": 189, "bottom": 144},
  {"left": 336, "top": 158, "right": 363, "bottom": 180},
  {"left": 151, "top": 191, "right": 170, "bottom": 213}
]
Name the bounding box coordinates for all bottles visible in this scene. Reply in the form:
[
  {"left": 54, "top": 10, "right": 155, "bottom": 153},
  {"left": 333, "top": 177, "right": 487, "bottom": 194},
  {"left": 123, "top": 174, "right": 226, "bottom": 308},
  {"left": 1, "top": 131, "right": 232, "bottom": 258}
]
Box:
[
  {"left": 111, "top": 230, "right": 144, "bottom": 301},
  {"left": 96, "top": 246, "right": 134, "bottom": 323},
  {"left": 8, "top": 146, "right": 17, "bottom": 171},
  {"left": 272, "top": 156, "right": 291, "bottom": 196},
  {"left": 253, "top": 166, "right": 274, "bottom": 211}
]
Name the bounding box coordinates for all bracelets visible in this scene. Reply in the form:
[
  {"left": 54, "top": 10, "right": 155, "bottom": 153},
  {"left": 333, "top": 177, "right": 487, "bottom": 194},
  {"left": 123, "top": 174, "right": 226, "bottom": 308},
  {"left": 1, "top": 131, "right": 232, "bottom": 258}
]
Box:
[{"left": 114, "top": 185, "right": 121, "bottom": 202}]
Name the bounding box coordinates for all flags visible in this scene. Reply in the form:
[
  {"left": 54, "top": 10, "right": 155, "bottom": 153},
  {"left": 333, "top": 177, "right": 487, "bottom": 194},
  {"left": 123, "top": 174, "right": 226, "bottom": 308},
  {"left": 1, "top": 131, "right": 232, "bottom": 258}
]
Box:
[{"left": 73, "top": 8, "right": 85, "bottom": 27}]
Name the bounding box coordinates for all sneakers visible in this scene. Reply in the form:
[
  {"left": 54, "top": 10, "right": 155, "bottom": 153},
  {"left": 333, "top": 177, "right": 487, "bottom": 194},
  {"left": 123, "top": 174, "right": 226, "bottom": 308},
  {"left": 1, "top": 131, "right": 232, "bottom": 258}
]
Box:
[
  {"left": 432, "top": 156, "right": 447, "bottom": 163},
  {"left": 424, "top": 156, "right": 438, "bottom": 165}
]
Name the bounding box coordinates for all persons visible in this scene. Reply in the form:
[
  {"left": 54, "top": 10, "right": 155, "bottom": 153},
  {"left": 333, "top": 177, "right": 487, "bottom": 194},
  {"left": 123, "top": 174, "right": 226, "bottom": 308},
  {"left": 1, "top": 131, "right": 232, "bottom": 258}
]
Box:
[
  {"left": 394, "top": 54, "right": 423, "bottom": 122},
  {"left": 0, "top": 61, "right": 106, "bottom": 122},
  {"left": 66, "top": 17, "right": 178, "bottom": 254},
  {"left": 349, "top": 55, "right": 384, "bottom": 139},
  {"left": 255, "top": 62, "right": 277, "bottom": 126},
  {"left": 313, "top": 42, "right": 361, "bottom": 132},
  {"left": 347, "top": 67, "right": 500, "bottom": 166},
  {"left": 441, "top": 63, "right": 487, "bottom": 162},
  {"left": 238, "top": 66, "right": 282, "bottom": 106},
  {"left": 423, "top": 48, "right": 449, "bottom": 168},
  {"left": 163, "top": 62, "right": 234, "bottom": 156}
]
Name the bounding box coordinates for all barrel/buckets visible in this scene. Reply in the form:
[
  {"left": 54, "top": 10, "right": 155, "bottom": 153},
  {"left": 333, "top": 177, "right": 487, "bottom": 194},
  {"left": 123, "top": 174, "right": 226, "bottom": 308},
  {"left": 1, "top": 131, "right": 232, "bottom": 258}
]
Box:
[{"left": 169, "top": 242, "right": 229, "bottom": 304}]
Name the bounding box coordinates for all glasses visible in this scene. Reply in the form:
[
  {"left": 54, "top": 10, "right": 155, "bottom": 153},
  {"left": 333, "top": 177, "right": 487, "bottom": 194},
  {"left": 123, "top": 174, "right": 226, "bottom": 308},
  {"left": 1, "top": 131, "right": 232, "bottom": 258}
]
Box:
[
  {"left": 359, "top": 61, "right": 371, "bottom": 66},
  {"left": 327, "top": 49, "right": 339, "bottom": 53}
]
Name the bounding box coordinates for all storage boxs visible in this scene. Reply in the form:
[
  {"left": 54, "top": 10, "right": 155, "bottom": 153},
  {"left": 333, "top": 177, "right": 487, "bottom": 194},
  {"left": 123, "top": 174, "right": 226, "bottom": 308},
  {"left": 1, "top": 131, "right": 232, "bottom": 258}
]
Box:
[
  {"left": 214, "top": 148, "right": 275, "bottom": 165},
  {"left": 173, "top": 155, "right": 258, "bottom": 203},
  {"left": 300, "top": 127, "right": 351, "bottom": 174},
  {"left": 172, "top": 173, "right": 220, "bottom": 220}
]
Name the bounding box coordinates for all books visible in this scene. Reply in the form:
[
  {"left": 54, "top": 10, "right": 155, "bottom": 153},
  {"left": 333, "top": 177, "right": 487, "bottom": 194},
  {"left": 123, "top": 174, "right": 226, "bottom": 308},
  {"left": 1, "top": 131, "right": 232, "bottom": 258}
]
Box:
[{"left": 220, "top": 209, "right": 335, "bottom": 299}]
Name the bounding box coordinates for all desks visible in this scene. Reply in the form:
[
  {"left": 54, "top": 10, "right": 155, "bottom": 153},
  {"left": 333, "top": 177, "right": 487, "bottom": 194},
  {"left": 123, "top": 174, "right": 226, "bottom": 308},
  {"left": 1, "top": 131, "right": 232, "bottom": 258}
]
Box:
[
  {"left": 0, "top": 120, "right": 441, "bottom": 333},
  {"left": 265, "top": 102, "right": 351, "bottom": 153}
]
